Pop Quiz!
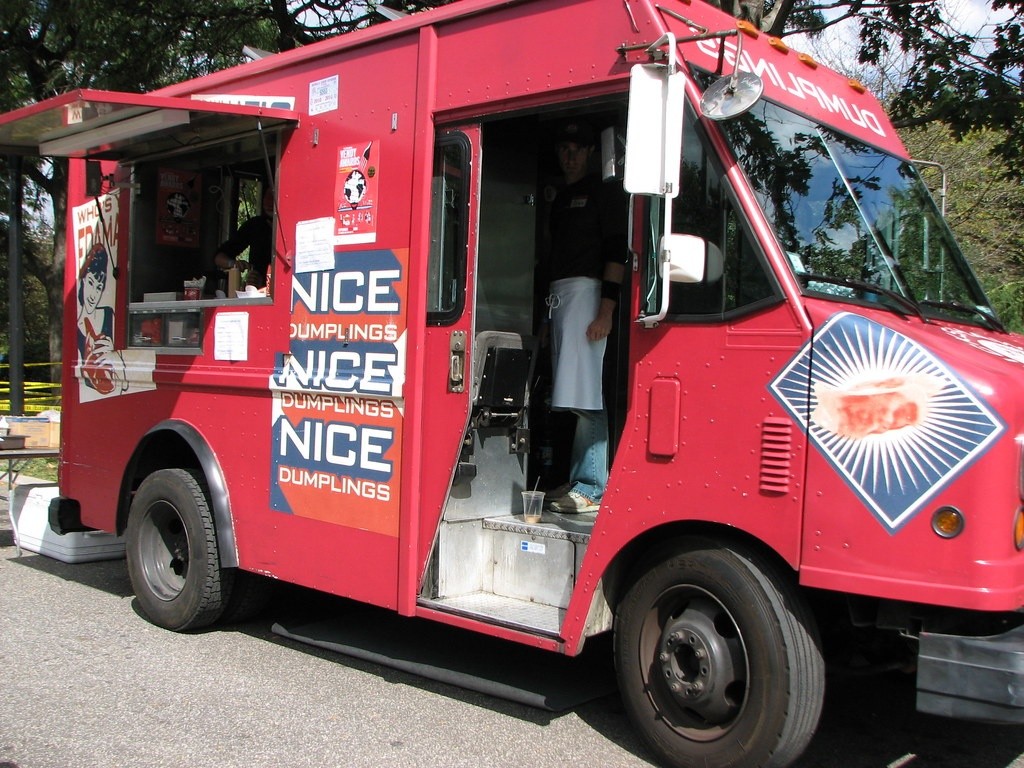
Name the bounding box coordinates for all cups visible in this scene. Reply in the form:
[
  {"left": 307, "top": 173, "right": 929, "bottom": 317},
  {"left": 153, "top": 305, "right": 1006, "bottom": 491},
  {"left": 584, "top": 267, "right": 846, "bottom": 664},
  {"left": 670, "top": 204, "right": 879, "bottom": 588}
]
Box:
[
  {"left": 521, "top": 490, "right": 546, "bottom": 524},
  {"left": 184, "top": 287, "right": 200, "bottom": 300}
]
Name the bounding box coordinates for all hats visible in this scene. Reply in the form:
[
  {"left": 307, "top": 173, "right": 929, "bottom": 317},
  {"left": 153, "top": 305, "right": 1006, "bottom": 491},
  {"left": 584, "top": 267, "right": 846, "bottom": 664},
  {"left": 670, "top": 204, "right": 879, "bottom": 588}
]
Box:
[{"left": 555, "top": 120, "right": 594, "bottom": 145}]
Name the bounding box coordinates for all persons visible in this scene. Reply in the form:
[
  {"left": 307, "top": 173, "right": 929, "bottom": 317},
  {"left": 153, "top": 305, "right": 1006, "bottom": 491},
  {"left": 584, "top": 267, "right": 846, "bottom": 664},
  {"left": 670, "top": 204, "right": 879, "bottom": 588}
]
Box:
[
  {"left": 213, "top": 184, "right": 273, "bottom": 290},
  {"left": 535, "top": 119, "right": 628, "bottom": 515}
]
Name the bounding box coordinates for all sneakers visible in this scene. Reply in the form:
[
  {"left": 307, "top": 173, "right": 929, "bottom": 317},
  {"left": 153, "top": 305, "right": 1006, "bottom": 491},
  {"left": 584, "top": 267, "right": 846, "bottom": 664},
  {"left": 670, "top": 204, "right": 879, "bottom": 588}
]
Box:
[
  {"left": 544, "top": 483, "right": 572, "bottom": 501},
  {"left": 550, "top": 490, "right": 600, "bottom": 513}
]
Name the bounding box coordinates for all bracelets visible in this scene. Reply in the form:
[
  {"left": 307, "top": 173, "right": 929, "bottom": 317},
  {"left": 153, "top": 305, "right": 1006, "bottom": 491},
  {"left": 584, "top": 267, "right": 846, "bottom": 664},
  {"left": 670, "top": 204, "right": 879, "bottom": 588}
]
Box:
[
  {"left": 227, "top": 259, "right": 233, "bottom": 268},
  {"left": 600, "top": 280, "right": 619, "bottom": 303}
]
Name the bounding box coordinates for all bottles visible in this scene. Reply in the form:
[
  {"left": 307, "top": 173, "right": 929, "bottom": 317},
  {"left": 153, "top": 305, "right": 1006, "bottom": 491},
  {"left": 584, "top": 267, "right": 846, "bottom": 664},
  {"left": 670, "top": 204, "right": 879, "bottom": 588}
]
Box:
[{"left": 228, "top": 264, "right": 240, "bottom": 298}]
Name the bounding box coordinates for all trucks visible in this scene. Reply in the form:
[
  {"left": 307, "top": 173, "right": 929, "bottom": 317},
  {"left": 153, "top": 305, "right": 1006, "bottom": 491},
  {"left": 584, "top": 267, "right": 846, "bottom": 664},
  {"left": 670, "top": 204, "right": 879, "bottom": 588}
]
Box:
[{"left": 0, "top": 0, "right": 1024, "bottom": 768}]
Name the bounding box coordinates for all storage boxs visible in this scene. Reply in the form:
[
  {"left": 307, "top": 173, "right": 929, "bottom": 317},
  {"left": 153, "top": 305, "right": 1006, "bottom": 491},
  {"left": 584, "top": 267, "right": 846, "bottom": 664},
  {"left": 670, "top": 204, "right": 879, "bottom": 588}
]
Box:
[
  {"left": 0, "top": 414, "right": 60, "bottom": 450},
  {"left": 13, "top": 483, "right": 126, "bottom": 564}
]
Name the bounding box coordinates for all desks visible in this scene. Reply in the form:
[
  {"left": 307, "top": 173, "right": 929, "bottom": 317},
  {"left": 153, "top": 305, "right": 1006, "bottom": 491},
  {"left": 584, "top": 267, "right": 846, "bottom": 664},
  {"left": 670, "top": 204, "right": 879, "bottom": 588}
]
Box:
[{"left": 0, "top": 447, "right": 61, "bottom": 557}]
